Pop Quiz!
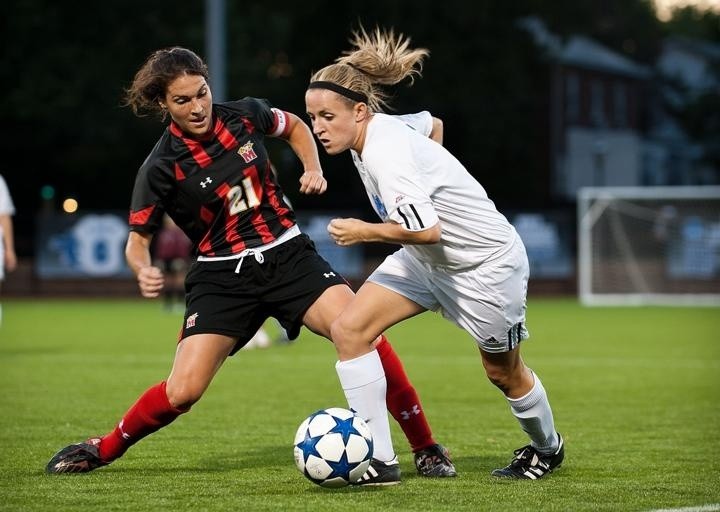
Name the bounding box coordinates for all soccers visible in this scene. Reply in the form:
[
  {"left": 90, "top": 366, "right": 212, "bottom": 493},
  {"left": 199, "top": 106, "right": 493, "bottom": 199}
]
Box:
[{"left": 294, "top": 408, "right": 373, "bottom": 488}]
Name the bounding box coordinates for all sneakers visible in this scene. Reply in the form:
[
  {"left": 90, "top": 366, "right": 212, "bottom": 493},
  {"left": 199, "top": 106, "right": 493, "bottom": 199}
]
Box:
[
  {"left": 349, "top": 454, "right": 402, "bottom": 488},
  {"left": 44, "top": 435, "right": 113, "bottom": 474},
  {"left": 414, "top": 442, "right": 457, "bottom": 477},
  {"left": 492, "top": 433, "right": 565, "bottom": 480}
]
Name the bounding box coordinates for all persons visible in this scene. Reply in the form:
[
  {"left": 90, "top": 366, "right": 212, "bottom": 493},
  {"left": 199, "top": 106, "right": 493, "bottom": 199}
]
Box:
[
  {"left": 155, "top": 211, "right": 193, "bottom": 305},
  {"left": 45, "top": 45, "right": 455, "bottom": 488},
  {"left": 0, "top": 176, "right": 18, "bottom": 283},
  {"left": 302, "top": 27, "right": 566, "bottom": 482}
]
